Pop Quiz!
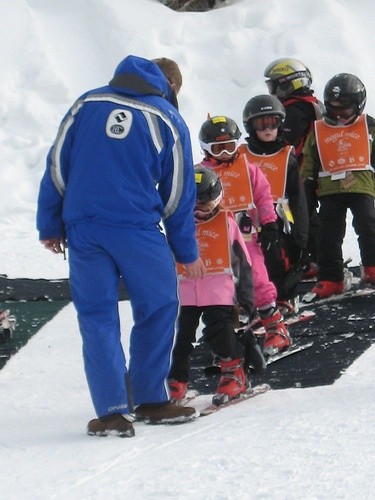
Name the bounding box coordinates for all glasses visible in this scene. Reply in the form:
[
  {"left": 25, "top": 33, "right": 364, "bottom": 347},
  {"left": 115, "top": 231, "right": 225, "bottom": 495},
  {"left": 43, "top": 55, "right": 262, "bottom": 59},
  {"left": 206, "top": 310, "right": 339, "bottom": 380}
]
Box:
[
  {"left": 247, "top": 114, "right": 286, "bottom": 131},
  {"left": 264, "top": 79, "right": 278, "bottom": 94},
  {"left": 325, "top": 99, "right": 360, "bottom": 120},
  {"left": 198, "top": 136, "right": 243, "bottom": 157}
]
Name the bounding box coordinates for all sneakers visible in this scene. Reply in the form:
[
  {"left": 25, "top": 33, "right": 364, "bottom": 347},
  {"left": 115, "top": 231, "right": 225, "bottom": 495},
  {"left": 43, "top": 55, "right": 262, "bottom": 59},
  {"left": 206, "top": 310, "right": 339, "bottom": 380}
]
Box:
[
  {"left": 301, "top": 262, "right": 318, "bottom": 281},
  {"left": 301, "top": 280, "right": 344, "bottom": 303},
  {"left": 213, "top": 356, "right": 250, "bottom": 406},
  {"left": 360, "top": 265, "right": 375, "bottom": 289},
  {"left": 256, "top": 303, "right": 293, "bottom": 358},
  {"left": 234, "top": 294, "right": 299, "bottom": 336},
  {"left": 167, "top": 379, "right": 189, "bottom": 405}
]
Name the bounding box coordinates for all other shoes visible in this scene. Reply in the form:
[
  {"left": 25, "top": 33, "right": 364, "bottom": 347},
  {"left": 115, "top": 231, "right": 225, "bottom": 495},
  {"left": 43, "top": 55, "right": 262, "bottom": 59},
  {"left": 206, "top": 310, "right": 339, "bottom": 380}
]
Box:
[
  {"left": 87, "top": 412, "right": 134, "bottom": 438},
  {"left": 133, "top": 400, "right": 198, "bottom": 425}
]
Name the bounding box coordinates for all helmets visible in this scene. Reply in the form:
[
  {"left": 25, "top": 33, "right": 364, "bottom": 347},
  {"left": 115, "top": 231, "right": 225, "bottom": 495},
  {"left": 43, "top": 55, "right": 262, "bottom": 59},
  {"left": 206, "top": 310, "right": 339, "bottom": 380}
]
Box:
[
  {"left": 323, "top": 72, "right": 366, "bottom": 125},
  {"left": 264, "top": 58, "right": 313, "bottom": 102},
  {"left": 242, "top": 94, "right": 287, "bottom": 124},
  {"left": 192, "top": 163, "right": 223, "bottom": 214},
  {"left": 199, "top": 113, "right": 242, "bottom": 144}
]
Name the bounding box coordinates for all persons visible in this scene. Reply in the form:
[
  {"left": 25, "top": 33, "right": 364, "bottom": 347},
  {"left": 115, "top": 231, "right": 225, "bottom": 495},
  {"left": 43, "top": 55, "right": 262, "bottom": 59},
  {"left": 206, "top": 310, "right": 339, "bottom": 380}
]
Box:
[
  {"left": 299, "top": 73, "right": 375, "bottom": 304},
  {"left": 263, "top": 57, "right": 327, "bottom": 279},
  {"left": 36, "top": 55, "right": 208, "bottom": 439},
  {"left": 237, "top": 94, "right": 310, "bottom": 329},
  {"left": 166, "top": 164, "right": 257, "bottom": 405},
  {"left": 194, "top": 116, "right": 293, "bottom": 357}
]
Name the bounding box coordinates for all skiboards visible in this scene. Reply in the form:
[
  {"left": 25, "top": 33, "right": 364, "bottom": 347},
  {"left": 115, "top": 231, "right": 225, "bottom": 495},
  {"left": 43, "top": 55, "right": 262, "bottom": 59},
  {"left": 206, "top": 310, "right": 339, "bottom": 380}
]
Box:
[
  {"left": 234, "top": 310, "right": 315, "bottom": 335},
  {"left": 301, "top": 276, "right": 361, "bottom": 285},
  {"left": 204, "top": 341, "right": 313, "bottom": 373},
  {"left": 172, "top": 383, "right": 271, "bottom": 416},
  {"left": 294, "top": 288, "right": 375, "bottom": 308}
]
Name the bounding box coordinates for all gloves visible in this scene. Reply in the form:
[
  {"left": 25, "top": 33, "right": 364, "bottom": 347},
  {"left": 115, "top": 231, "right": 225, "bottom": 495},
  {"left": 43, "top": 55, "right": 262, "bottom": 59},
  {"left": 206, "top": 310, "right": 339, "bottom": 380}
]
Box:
[{"left": 255, "top": 222, "right": 280, "bottom": 252}]
